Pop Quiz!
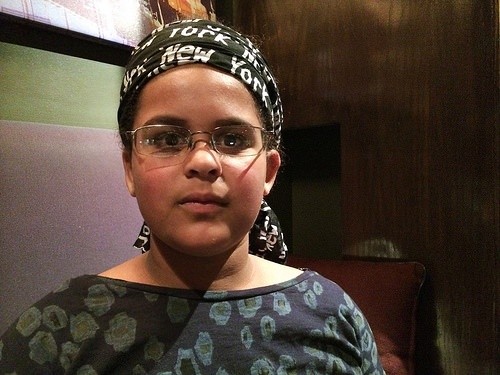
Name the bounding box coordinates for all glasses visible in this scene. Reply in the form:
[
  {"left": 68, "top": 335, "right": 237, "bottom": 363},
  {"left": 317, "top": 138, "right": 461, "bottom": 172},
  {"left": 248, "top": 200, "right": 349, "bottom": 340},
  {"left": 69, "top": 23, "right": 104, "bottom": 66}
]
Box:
[{"left": 125, "top": 124, "right": 278, "bottom": 159}]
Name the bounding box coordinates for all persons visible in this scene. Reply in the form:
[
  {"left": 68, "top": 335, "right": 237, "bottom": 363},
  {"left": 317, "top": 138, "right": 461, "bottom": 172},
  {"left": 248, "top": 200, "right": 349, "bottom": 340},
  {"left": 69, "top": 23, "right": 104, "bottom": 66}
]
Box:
[{"left": 1, "top": 19, "right": 387, "bottom": 375}]
